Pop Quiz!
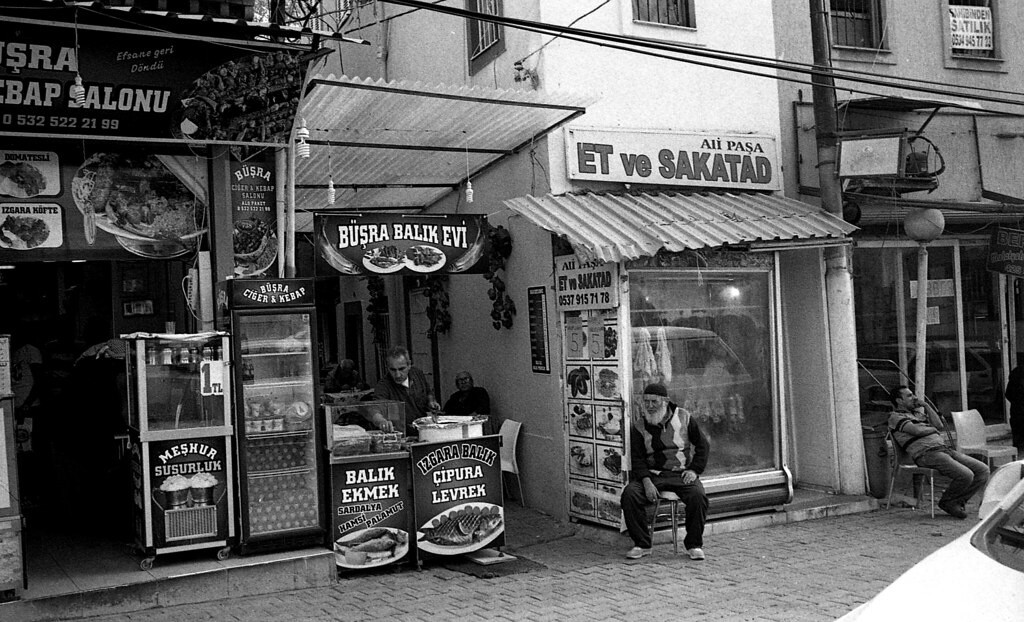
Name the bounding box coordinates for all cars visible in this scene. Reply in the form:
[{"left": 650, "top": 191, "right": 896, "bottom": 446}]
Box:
[
  {"left": 830, "top": 460, "right": 1024, "bottom": 622},
  {"left": 859, "top": 340, "right": 1000, "bottom": 407}
]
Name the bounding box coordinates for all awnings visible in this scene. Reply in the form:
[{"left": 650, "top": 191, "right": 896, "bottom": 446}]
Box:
[
  {"left": 287, "top": 76, "right": 601, "bottom": 213},
  {"left": 843, "top": 96, "right": 984, "bottom": 109},
  {"left": 503, "top": 192, "right": 860, "bottom": 261}
]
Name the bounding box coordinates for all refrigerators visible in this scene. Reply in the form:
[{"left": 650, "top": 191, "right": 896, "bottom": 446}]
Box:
[{"left": 215, "top": 277, "right": 328, "bottom": 558}]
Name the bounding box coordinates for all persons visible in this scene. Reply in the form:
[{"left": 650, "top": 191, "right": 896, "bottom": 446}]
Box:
[
  {"left": 443, "top": 371, "right": 491, "bottom": 415},
  {"left": 325, "top": 359, "right": 359, "bottom": 394},
  {"left": 889, "top": 386, "right": 989, "bottom": 519},
  {"left": 366, "top": 345, "right": 441, "bottom": 434},
  {"left": 1006, "top": 363, "right": 1024, "bottom": 448},
  {"left": 621, "top": 384, "right": 708, "bottom": 559}
]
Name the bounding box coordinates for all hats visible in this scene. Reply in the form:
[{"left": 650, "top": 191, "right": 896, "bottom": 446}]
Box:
[{"left": 644, "top": 384, "right": 668, "bottom": 397}]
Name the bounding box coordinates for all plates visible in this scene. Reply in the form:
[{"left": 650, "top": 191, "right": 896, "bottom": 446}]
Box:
[
  {"left": 417, "top": 501, "right": 505, "bottom": 555},
  {"left": 71, "top": 156, "right": 208, "bottom": 241},
  {"left": 333, "top": 527, "right": 408, "bottom": 568},
  {"left": 362, "top": 244, "right": 446, "bottom": 273},
  {"left": 0, "top": 176, "right": 48, "bottom": 199},
  {"left": 234, "top": 219, "right": 277, "bottom": 275},
  {"left": 0, "top": 217, "right": 50, "bottom": 250}
]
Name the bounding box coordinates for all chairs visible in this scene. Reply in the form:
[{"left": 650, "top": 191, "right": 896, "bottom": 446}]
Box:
[
  {"left": 951, "top": 409, "right": 1018, "bottom": 474},
  {"left": 885, "top": 428, "right": 949, "bottom": 518},
  {"left": 499, "top": 419, "right": 527, "bottom": 507},
  {"left": 650, "top": 491, "right": 682, "bottom": 555}
]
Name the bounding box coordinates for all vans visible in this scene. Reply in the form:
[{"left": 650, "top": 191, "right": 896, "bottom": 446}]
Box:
[{"left": 627, "top": 326, "right": 754, "bottom": 443}]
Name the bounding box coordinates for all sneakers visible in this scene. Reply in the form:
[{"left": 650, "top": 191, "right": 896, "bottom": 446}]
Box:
[
  {"left": 686, "top": 546, "right": 706, "bottom": 560},
  {"left": 626, "top": 547, "right": 652, "bottom": 559}
]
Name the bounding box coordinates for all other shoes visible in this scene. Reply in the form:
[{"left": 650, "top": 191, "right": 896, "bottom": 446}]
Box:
[{"left": 938, "top": 500, "right": 968, "bottom": 518}]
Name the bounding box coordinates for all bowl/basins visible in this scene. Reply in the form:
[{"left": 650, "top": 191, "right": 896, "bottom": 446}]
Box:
[
  {"left": 413, "top": 415, "right": 488, "bottom": 442},
  {"left": 234, "top": 235, "right": 267, "bottom": 261}
]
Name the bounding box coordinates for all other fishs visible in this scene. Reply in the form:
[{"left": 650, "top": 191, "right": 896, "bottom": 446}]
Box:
[
  {"left": 334, "top": 528, "right": 398, "bottom": 553},
  {"left": 418, "top": 513, "right": 503, "bottom": 544}
]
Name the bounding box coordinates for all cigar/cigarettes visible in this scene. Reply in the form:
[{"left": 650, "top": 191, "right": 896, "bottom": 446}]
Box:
[{"left": 652, "top": 499, "right": 656, "bottom": 502}]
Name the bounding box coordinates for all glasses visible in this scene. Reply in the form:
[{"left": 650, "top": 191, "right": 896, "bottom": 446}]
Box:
[{"left": 456, "top": 377, "right": 470, "bottom": 384}]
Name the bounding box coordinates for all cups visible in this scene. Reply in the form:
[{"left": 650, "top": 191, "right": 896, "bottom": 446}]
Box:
[
  {"left": 244, "top": 419, "right": 283, "bottom": 433},
  {"left": 250, "top": 404, "right": 261, "bottom": 417},
  {"left": 165, "top": 322, "right": 176, "bottom": 334},
  {"left": 160, "top": 486, "right": 191, "bottom": 505},
  {"left": 189, "top": 485, "right": 215, "bottom": 502}
]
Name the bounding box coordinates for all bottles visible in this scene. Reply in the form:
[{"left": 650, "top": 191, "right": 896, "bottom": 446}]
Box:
[
  {"left": 245, "top": 435, "right": 308, "bottom": 471},
  {"left": 241, "top": 333, "right": 249, "bottom": 354},
  {"left": 248, "top": 477, "right": 317, "bottom": 532},
  {"left": 147, "top": 345, "right": 223, "bottom": 364},
  {"left": 243, "top": 357, "right": 255, "bottom": 383}
]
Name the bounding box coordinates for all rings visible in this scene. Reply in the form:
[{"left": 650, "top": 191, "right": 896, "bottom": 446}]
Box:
[{"left": 383, "top": 425, "right": 385, "bottom": 428}]
